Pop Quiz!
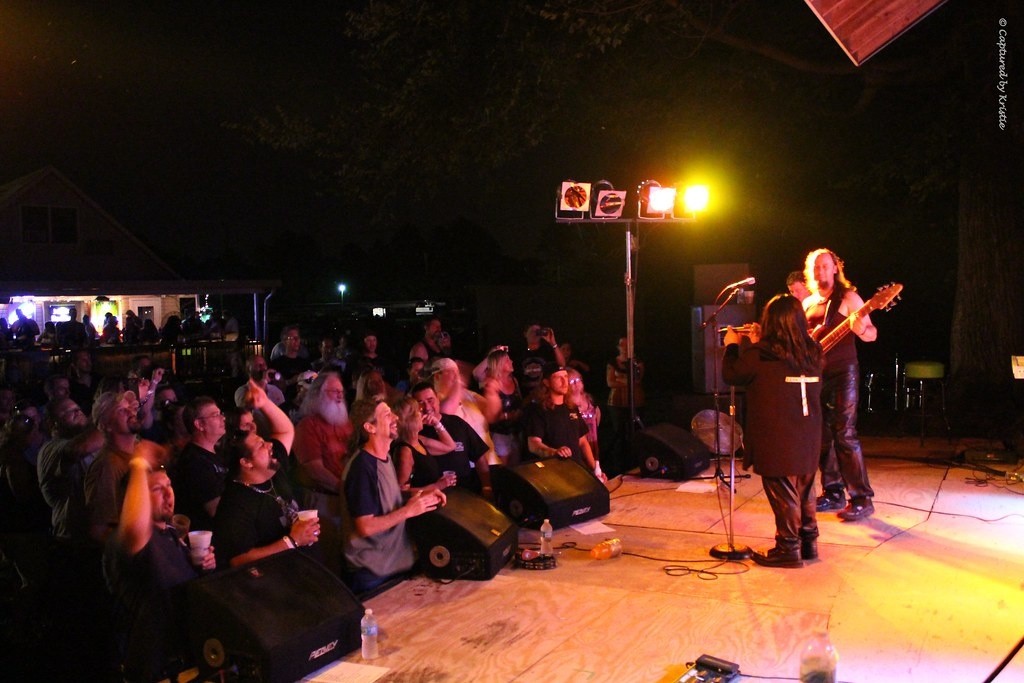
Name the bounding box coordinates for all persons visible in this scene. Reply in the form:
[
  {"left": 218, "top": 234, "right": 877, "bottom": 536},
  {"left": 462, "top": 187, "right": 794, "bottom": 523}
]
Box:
[
  {"left": 787, "top": 248, "right": 878, "bottom": 520},
  {"left": 722, "top": 294, "right": 826, "bottom": 568},
  {"left": 0, "top": 308, "right": 647, "bottom": 683}
]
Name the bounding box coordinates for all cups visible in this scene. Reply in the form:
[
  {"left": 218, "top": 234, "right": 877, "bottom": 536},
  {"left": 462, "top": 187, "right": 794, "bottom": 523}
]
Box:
[
  {"left": 188, "top": 531, "right": 213, "bottom": 565},
  {"left": 297, "top": 510, "right": 318, "bottom": 535},
  {"left": 443, "top": 471, "right": 456, "bottom": 485}
]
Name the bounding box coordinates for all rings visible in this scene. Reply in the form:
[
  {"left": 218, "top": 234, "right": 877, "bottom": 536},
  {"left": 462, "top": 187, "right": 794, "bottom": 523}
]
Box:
[{"left": 314, "top": 529, "right": 319, "bottom": 536}]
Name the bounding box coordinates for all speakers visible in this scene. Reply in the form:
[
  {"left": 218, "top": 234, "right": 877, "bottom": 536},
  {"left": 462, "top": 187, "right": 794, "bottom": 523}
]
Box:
[
  {"left": 668, "top": 384, "right": 744, "bottom": 459},
  {"left": 178, "top": 547, "right": 366, "bottom": 683},
  {"left": 692, "top": 303, "right": 756, "bottom": 395},
  {"left": 497, "top": 453, "right": 611, "bottom": 530},
  {"left": 404, "top": 487, "right": 519, "bottom": 581},
  {"left": 632, "top": 421, "right": 710, "bottom": 481}
]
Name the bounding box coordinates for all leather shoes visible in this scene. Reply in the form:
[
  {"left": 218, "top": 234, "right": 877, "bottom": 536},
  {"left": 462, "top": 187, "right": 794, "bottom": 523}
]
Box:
[
  {"left": 816, "top": 491, "right": 846, "bottom": 512},
  {"left": 838, "top": 496, "right": 874, "bottom": 521},
  {"left": 751, "top": 544, "right": 802, "bottom": 567},
  {"left": 802, "top": 541, "right": 817, "bottom": 559}
]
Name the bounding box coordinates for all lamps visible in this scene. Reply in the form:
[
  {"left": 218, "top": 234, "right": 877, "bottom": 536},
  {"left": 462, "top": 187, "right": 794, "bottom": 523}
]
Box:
[{"left": 552, "top": 175, "right": 677, "bottom": 220}]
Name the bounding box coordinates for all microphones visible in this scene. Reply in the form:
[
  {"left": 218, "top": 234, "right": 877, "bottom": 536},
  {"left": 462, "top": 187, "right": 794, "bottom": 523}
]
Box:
[{"left": 727, "top": 277, "right": 755, "bottom": 290}]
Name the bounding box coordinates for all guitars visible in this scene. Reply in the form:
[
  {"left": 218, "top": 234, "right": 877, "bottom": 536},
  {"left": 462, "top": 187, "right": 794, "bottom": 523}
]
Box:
[{"left": 811, "top": 281, "right": 906, "bottom": 374}]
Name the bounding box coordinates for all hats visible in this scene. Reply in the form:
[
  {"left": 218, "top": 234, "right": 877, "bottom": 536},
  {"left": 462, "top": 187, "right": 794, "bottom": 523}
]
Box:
[
  {"left": 92, "top": 390, "right": 136, "bottom": 424},
  {"left": 430, "top": 358, "right": 458, "bottom": 375},
  {"left": 543, "top": 361, "right": 566, "bottom": 380},
  {"left": 350, "top": 398, "right": 383, "bottom": 443}
]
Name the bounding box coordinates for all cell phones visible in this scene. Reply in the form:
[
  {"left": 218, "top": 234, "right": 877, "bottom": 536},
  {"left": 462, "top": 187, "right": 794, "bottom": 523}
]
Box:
[{"left": 535, "top": 329, "right": 549, "bottom": 336}]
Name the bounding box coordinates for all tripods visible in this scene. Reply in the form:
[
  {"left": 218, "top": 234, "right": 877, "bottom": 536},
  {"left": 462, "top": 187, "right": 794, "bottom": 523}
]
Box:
[{"left": 685, "top": 288, "right": 751, "bottom": 494}]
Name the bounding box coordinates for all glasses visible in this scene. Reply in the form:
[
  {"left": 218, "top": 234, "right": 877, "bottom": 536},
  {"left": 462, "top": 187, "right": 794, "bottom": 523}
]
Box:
[
  {"left": 569, "top": 378, "right": 581, "bottom": 384},
  {"left": 327, "top": 388, "right": 345, "bottom": 395},
  {"left": 200, "top": 412, "right": 224, "bottom": 420}
]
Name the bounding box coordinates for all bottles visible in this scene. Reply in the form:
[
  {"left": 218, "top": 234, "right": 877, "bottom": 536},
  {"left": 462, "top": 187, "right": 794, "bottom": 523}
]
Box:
[
  {"left": 361, "top": 609, "right": 378, "bottom": 660},
  {"left": 540, "top": 519, "right": 553, "bottom": 555},
  {"left": 590, "top": 538, "right": 622, "bottom": 560},
  {"left": 262, "top": 371, "right": 281, "bottom": 381},
  {"left": 535, "top": 329, "right": 548, "bottom": 336}
]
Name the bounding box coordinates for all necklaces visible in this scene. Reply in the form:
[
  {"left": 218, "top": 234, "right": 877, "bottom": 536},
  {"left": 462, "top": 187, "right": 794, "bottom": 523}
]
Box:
[{"left": 231, "top": 478, "right": 289, "bottom": 507}]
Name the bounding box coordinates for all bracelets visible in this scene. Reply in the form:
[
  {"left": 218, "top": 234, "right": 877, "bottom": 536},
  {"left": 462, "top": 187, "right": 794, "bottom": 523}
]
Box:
[
  {"left": 858, "top": 325, "right": 874, "bottom": 339},
  {"left": 127, "top": 456, "right": 151, "bottom": 471},
  {"left": 151, "top": 379, "right": 159, "bottom": 385},
  {"left": 261, "top": 378, "right": 269, "bottom": 384},
  {"left": 282, "top": 535, "right": 297, "bottom": 549},
  {"left": 552, "top": 344, "right": 558, "bottom": 348},
  {"left": 504, "top": 412, "right": 508, "bottom": 421},
  {"left": 433, "top": 421, "right": 445, "bottom": 433}
]
